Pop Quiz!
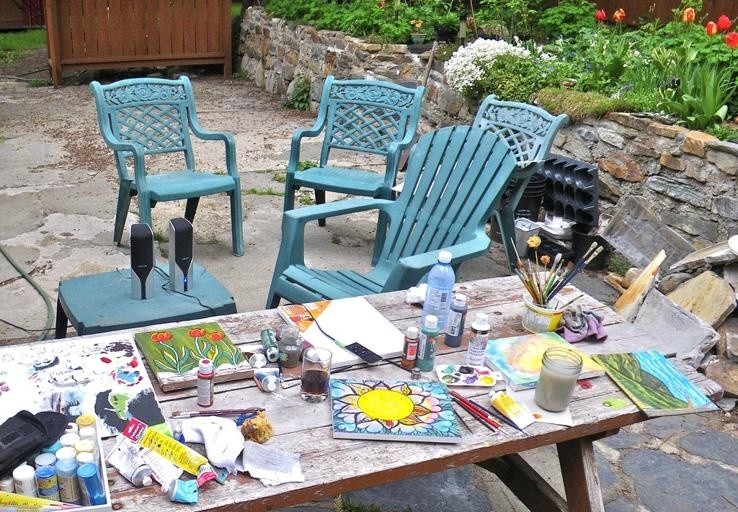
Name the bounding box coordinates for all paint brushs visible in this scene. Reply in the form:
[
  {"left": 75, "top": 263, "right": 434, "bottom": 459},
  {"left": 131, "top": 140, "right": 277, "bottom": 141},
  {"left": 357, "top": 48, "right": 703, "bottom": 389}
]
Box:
[
  {"left": 506, "top": 235, "right": 606, "bottom": 315},
  {"left": 491, "top": 417, "right": 503, "bottom": 427},
  {"left": 449, "top": 395, "right": 496, "bottom": 434},
  {"left": 168, "top": 412, "right": 227, "bottom": 418},
  {"left": 469, "top": 398, "right": 533, "bottom": 437},
  {"left": 452, "top": 396, "right": 510, "bottom": 436},
  {"left": 448, "top": 389, "right": 492, "bottom": 418},
  {"left": 282, "top": 366, "right": 353, "bottom": 389},
  {"left": 172, "top": 410, "right": 258, "bottom": 415},
  {"left": 453, "top": 407, "right": 474, "bottom": 435}
]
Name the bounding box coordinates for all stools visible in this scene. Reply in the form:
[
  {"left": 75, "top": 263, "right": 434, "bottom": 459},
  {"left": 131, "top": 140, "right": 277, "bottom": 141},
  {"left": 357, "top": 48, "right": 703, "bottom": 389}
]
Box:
[{"left": 57, "top": 263, "right": 238, "bottom": 339}]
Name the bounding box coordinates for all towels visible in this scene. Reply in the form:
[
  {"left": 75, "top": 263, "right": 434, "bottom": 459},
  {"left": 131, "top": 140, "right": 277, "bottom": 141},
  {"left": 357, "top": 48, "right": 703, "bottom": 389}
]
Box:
[
  {"left": 150, "top": 414, "right": 243, "bottom": 488},
  {"left": 561, "top": 303, "right": 607, "bottom": 344}
]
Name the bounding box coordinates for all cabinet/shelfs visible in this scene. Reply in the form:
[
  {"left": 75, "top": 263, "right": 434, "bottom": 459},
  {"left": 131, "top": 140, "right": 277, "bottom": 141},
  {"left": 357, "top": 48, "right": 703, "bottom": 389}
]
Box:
[{"left": 44, "top": 0, "right": 232, "bottom": 90}]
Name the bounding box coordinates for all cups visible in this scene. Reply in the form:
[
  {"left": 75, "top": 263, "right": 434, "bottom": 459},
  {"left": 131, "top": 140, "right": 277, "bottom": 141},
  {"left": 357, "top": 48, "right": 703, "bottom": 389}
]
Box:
[
  {"left": 522, "top": 291, "right": 569, "bottom": 334},
  {"left": 535, "top": 347, "right": 583, "bottom": 412},
  {"left": 300, "top": 347, "right": 332, "bottom": 403}
]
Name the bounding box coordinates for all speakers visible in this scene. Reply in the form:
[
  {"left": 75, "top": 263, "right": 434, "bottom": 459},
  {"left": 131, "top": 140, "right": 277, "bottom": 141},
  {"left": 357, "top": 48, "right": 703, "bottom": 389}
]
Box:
[
  {"left": 168, "top": 217, "right": 194, "bottom": 293},
  {"left": 129, "top": 223, "right": 156, "bottom": 299}
]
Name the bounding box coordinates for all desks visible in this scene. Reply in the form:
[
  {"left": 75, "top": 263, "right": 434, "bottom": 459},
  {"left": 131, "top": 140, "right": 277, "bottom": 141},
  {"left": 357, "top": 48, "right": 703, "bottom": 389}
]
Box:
[{"left": 0, "top": 269, "right": 723, "bottom": 512}]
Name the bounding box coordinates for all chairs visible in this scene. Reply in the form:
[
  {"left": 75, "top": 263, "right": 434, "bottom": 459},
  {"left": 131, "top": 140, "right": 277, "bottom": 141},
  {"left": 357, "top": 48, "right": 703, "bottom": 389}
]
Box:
[
  {"left": 280, "top": 74, "right": 426, "bottom": 228},
  {"left": 267, "top": 123, "right": 516, "bottom": 309},
  {"left": 91, "top": 74, "right": 244, "bottom": 257},
  {"left": 472, "top": 94, "right": 570, "bottom": 276}
]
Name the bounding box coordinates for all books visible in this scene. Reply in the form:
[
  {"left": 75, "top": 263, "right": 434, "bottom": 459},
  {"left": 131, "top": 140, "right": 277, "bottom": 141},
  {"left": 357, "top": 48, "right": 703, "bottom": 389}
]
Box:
[
  {"left": 277, "top": 297, "right": 406, "bottom": 370},
  {"left": 327, "top": 377, "right": 462, "bottom": 445},
  {"left": 134, "top": 322, "right": 253, "bottom": 392},
  {"left": 485, "top": 333, "right": 606, "bottom": 392},
  {"left": 591, "top": 350, "right": 721, "bottom": 417}
]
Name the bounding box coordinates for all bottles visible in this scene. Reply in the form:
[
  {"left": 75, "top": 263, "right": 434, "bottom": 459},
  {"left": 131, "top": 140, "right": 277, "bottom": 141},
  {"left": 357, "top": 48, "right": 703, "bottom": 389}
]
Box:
[
  {"left": 466, "top": 312, "right": 491, "bottom": 365},
  {"left": 411, "top": 367, "right": 421, "bottom": 379},
  {"left": 444, "top": 293, "right": 468, "bottom": 347},
  {"left": 197, "top": 358, "right": 214, "bottom": 407},
  {"left": 416, "top": 314, "right": 439, "bottom": 371},
  {"left": 260, "top": 329, "right": 279, "bottom": 362},
  {"left": 401, "top": 326, "right": 419, "bottom": 368},
  {"left": 420, "top": 251, "right": 455, "bottom": 330}
]
